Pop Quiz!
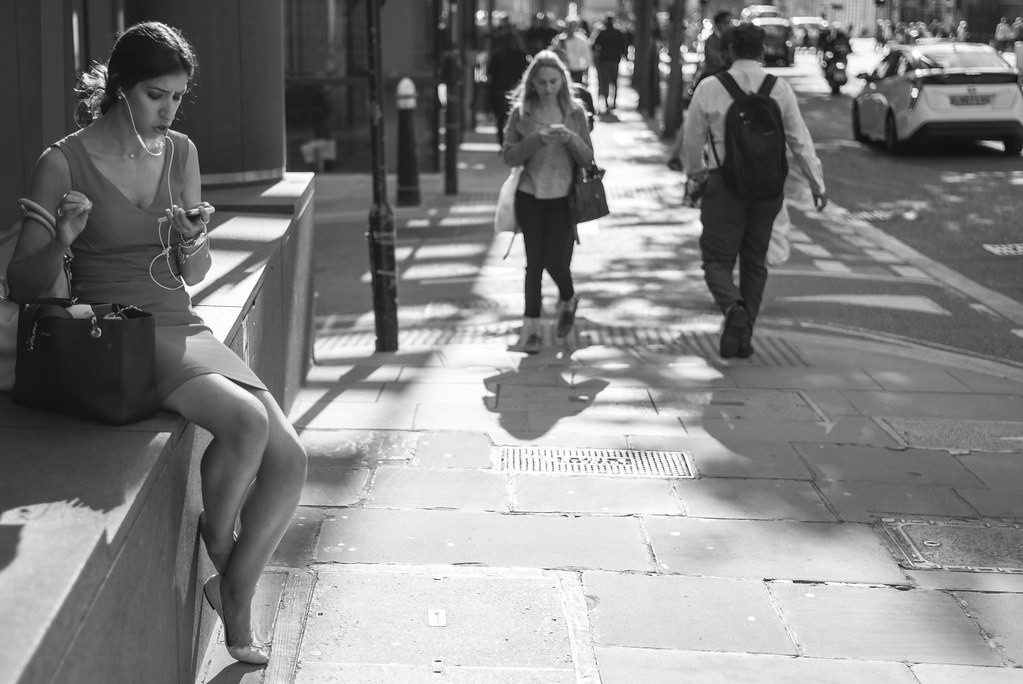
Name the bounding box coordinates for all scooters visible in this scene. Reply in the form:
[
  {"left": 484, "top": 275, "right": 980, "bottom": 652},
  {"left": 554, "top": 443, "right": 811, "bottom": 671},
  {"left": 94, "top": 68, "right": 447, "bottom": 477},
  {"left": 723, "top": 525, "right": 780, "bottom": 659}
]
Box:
[{"left": 821, "top": 44, "right": 848, "bottom": 93}]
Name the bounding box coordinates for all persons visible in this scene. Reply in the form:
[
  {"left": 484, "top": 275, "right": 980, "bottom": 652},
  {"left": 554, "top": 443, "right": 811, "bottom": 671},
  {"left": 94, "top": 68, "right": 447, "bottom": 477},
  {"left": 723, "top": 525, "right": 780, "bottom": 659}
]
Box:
[
  {"left": 995, "top": 16, "right": 1023, "bottom": 57},
  {"left": 6, "top": 22, "right": 308, "bottom": 664},
  {"left": 528, "top": 15, "right": 557, "bottom": 63},
  {"left": 589, "top": 11, "right": 629, "bottom": 112},
  {"left": 798, "top": 26, "right": 809, "bottom": 51},
  {"left": 683, "top": 23, "right": 829, "bottom": 357},
  {"left": 822, "top": 22, "right": 851, "bottom": 78},
  {"left": 668, "top": 11, "right": 733, "bottom": 170},
  {"left": 816, "top": 22, "right": 831, "bottom": 54},
  {"left": 551, "top": 20, "right": 592, "bottom": 90},
  {"left": 504, "top": 50, "right": 594, "bottom": 353},
  {"left": 874, "top": 18, "right": 969, "bottom": 53},
  {"left": 487, "top": 25, "right": 526, "bottom": 153}
]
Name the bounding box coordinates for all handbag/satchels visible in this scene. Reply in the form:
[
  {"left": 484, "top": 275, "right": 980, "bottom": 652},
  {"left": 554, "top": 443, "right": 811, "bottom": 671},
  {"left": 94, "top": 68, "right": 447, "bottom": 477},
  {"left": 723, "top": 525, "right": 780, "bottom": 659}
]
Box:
[
  {"left": 566, "top": 160, "right": 611, "bottom": 224},
  {"left": 13, "top": 197, "right": 156, "bottom": 425},
  {"left": 493, "top": 164, "right": 524, "bottom": 235}
]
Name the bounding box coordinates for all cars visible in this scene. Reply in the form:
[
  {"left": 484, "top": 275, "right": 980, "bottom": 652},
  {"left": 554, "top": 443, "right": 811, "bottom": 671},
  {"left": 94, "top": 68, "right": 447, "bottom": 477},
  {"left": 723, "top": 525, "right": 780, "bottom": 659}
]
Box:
[
  {"left": 741, "top": 3, "right": 827, "bottom": 67},
  {"left": 852, "top": 44, "right": 1023, "bottom": 156}
]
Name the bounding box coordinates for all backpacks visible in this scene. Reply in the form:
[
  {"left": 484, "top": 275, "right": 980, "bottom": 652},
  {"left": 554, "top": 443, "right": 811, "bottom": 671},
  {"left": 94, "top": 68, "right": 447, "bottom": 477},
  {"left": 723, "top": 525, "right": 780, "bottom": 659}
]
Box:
[{"left": 714, "top": 70, "right": 789, "bottom": 198}]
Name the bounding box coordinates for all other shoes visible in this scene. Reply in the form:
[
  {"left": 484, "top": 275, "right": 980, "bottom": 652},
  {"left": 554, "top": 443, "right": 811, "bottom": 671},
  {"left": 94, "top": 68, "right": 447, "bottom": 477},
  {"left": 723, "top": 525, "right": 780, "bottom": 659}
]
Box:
[
  {"left": 720, "top": 303, "right": 751, "bottom": 357},
  {"left": 203, "top": 572, "right": 270, "bottom": 662},
  {"left": 558, "top": 295, "right": 578, "bottom": 338},
  {"left": 525, "top": 334, "right": 542, "bottom": 353},
  {"left": 199, "top": 508, "right": 241, "bottom": 572},
  {"left": 737, "top": 344, "right": 754, "bottom": 357}
]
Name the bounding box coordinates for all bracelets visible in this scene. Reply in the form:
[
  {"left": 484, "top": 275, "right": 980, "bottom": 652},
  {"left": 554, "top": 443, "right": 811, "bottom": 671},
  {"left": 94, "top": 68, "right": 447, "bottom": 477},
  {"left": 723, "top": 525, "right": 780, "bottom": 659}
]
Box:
[{"left": 177, "top": 227, "right": 212, "bottom": 264}]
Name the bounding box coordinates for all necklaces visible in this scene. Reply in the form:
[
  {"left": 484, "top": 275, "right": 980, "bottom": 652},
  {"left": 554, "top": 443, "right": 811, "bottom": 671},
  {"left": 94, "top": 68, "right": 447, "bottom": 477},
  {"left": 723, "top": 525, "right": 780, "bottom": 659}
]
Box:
[{"left": 101, "top": 118, "right": 135, "bottom": 159}]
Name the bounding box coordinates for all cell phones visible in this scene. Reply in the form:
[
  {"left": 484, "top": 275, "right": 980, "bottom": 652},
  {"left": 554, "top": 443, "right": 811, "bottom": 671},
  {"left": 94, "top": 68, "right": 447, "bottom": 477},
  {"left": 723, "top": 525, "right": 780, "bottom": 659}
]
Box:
[
  {"left": 550, "top": 124, "right": 565, "bottom": 134},
  {"left": 157, "top": 207, "right": 216, "bottom": 223}
]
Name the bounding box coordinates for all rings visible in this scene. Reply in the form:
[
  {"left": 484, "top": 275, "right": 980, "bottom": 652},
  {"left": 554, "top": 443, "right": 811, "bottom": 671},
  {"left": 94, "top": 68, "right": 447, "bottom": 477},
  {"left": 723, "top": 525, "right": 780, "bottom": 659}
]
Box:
[
  {"left": 57, "top": 209, "right": 65, "bottom": 217},
  {"left": 200, "top": 218, "right": 211, "bottom": 226}
]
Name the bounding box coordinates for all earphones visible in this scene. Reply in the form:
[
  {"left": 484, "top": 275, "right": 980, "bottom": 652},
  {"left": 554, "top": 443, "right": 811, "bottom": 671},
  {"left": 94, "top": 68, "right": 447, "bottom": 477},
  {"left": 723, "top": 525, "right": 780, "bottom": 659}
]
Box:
[{"left": 118, "top": 88, "right": 125, "bottom": 99}]
